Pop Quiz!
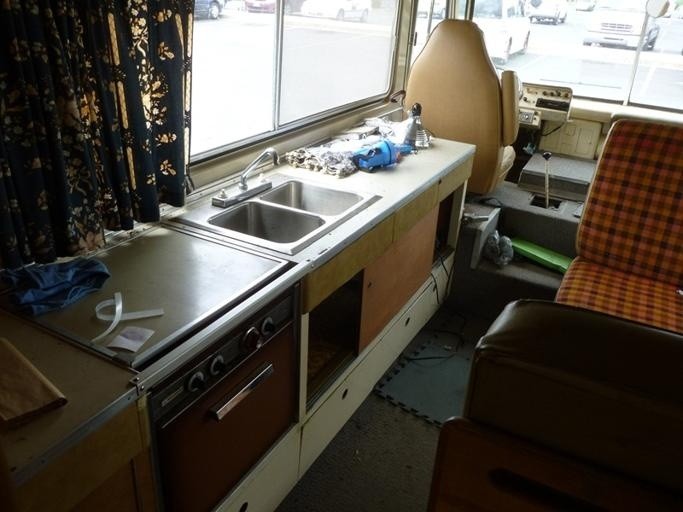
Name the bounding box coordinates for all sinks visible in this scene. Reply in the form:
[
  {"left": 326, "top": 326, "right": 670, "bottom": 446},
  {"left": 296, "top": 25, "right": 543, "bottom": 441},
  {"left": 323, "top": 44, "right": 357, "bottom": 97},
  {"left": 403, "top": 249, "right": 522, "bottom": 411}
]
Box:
[
  {"left": 162, "top": 200, "right": 328, "bottom": 255},
  {"left": 257, "top": 173, "right": 384, "bottom": 220}
]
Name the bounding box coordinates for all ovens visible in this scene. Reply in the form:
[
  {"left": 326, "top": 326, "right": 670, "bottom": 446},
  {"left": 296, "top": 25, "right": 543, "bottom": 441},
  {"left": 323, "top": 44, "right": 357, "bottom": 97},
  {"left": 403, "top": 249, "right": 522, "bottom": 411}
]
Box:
[{"left": 150, "top": 324, "right": 300, "bottom": 512}]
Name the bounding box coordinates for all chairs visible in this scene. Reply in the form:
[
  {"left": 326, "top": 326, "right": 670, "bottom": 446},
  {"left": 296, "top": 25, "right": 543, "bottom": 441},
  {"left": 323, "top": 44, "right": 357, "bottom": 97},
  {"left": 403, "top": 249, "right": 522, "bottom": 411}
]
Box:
[
  {"left": 425, "top": 299, "right": 682, "bottom": 511},
  {"left": 555, "top": 120, "right": 681, "bottom": 335},
  {"left": 406, "top": 22, "right": 522, "bottom": 191}
]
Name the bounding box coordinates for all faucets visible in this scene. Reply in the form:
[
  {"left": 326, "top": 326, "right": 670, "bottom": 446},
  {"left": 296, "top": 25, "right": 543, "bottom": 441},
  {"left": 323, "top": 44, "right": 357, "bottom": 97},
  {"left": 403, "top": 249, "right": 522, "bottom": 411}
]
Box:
[{"left": 239, "top": 147, "right": 281, "bottom": 188}]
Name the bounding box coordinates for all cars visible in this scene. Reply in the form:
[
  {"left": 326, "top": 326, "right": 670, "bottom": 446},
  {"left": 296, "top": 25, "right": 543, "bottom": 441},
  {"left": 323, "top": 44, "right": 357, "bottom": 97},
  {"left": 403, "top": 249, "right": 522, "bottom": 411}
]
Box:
[
  {"left": 194, "top": 0, "right": 373, "bottom": 22},
  {"left": 466, "top": 0, "right": 662, "bottom": 73},
  {"left": 418, "top": 0, "right": 447, "bottom": 19}
]
